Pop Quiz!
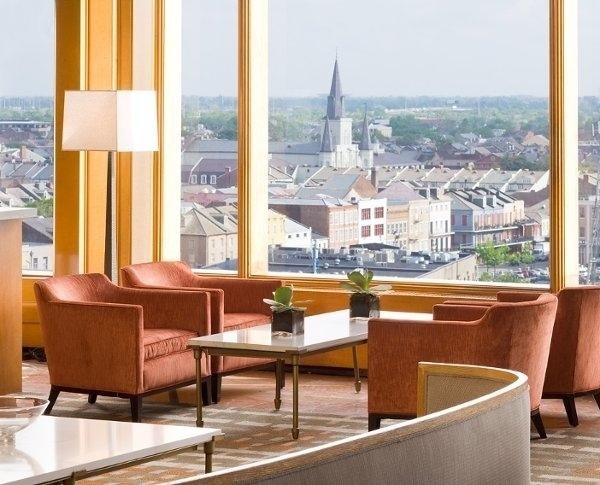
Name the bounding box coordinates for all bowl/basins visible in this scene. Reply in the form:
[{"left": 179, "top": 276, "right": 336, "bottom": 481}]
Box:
[{"left": 0, "top": 396, "right": 52, "bottom": 438}]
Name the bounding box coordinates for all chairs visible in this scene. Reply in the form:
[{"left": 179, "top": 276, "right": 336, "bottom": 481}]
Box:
[
  {"left": 362, "top": 286, "right": 600, "bottom": 441},
  {"left": 34, "top": 273, "right": 214, "bottom": 423},
  {"left": 149, "top": 358, "right": 534, "bottom": 485},
  {"left": 122, "top": 258, "right": 287, "bottom": 410}
]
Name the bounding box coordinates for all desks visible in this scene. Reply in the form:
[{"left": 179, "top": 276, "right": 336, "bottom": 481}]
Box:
[{"left": 2, "top": 415, "right": 222, "bottom": 485}]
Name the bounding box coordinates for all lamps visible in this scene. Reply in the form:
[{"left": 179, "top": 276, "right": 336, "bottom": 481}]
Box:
[{"left": 60, "top": 87, "right": 160, "bottom": 285}]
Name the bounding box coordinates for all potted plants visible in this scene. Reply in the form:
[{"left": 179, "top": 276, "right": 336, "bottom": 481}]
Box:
[
  {"left": 339, "top": 266, "right": 394, "bottom": 319},
  {"left": 263, "top": 282, "right": 308, "bottom": 336}
]
